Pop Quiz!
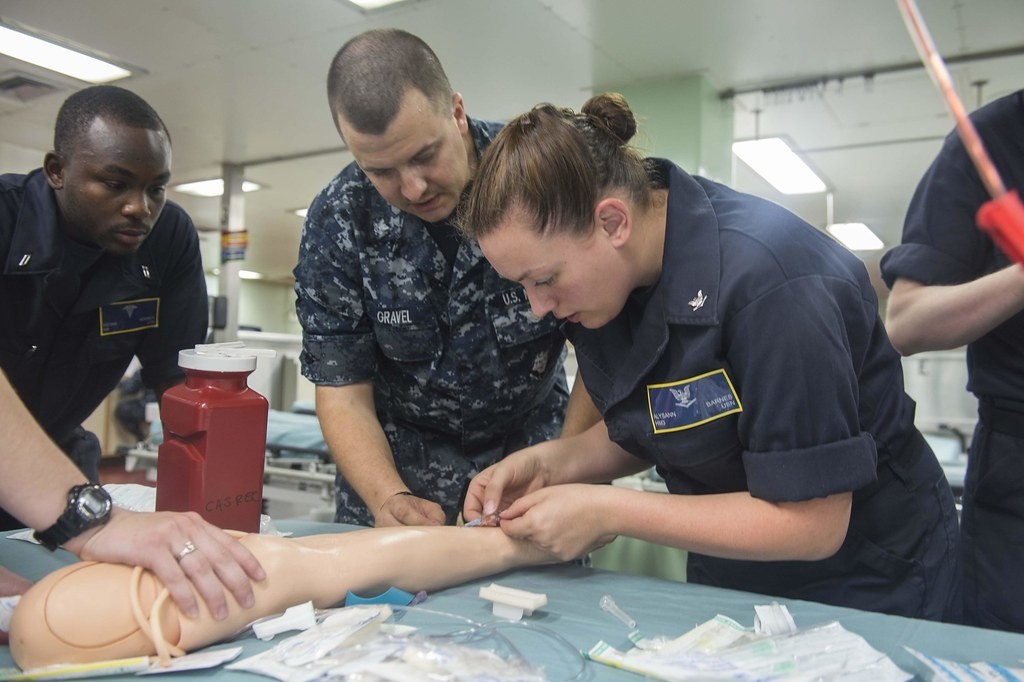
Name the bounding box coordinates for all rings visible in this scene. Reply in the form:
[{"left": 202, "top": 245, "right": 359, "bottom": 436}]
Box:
[{"left": 174, "top": 539, "right": 196, "bottom": 563}]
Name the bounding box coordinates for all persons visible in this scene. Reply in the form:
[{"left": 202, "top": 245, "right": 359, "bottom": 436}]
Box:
[
  {"left": 294, "top": 28, "right": 569, "bottom": 527},
  {"left": 113, "top": 366, "right": 156, "bottom": 445},
  {"left": 10, "top": 525, "right": 590, "bottom": 671},
  {"left": 461, "top": 93, "right": 960, "bottom": 628},
  {"left": 0, "top": 372, "right": 266, "bottom": 644},
  {"left": 0, "top": 87, "right": 211, "bottom": 526},
  {"left": 881, "top": 90, "right": 1024, "bottom": 633}
]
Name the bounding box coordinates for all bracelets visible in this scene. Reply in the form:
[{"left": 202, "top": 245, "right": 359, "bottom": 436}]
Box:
[{"left": 380, "top": 491, "right": 415, "bottom": 511}]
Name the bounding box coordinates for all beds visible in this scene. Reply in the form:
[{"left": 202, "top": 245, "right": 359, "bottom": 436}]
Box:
[{"left": 149, "top": 401, "right": 328, "bottom": 491}]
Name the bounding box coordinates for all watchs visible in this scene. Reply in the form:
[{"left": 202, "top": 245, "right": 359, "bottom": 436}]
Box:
[{"left": 33, "top": 481, "right": 112, "bottom": 552}]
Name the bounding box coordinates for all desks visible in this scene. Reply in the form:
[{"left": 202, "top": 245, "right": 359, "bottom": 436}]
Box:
[{"left": 0, "top": 517, "right": 1024, "bottom": 682}]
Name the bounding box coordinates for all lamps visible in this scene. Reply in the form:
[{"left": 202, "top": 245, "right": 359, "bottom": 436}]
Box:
[{"left": 732, "top": 109, "right": 829, "bottom": 195}]
